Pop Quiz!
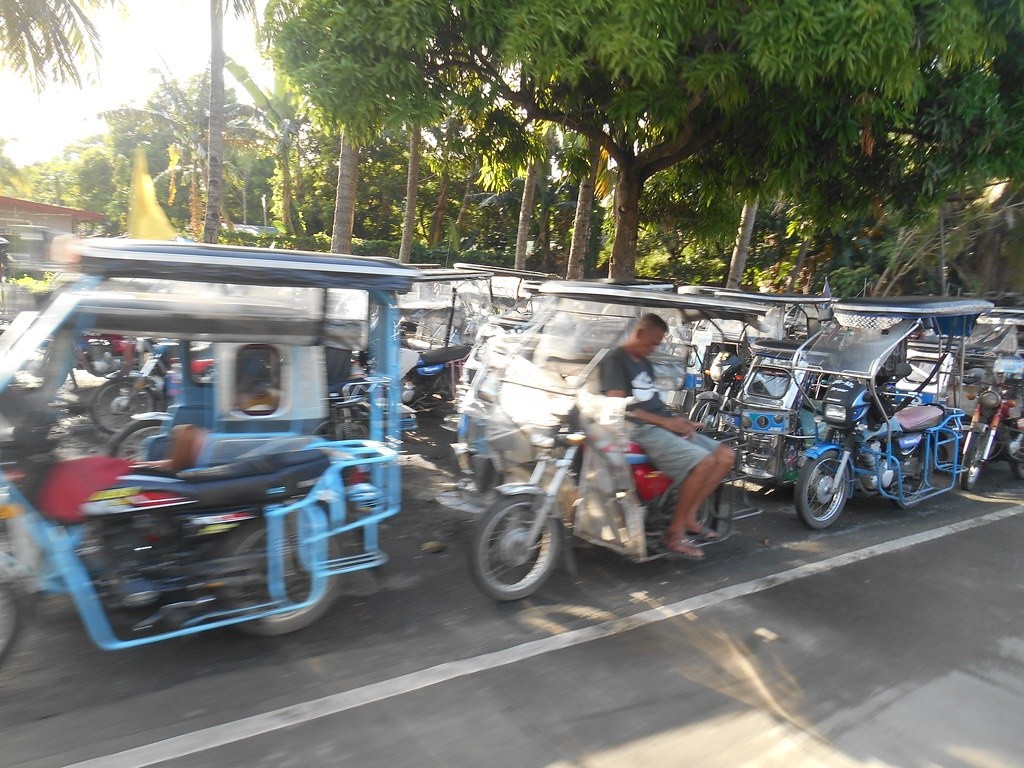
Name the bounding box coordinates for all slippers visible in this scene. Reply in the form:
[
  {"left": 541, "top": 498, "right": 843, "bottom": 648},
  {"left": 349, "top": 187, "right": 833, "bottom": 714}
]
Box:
[
  {"left": 662, "top": 536, "right": 705, "bottom": 562},
  {"left": 688, "top": 524, "right": 722, "bottom": 542}
]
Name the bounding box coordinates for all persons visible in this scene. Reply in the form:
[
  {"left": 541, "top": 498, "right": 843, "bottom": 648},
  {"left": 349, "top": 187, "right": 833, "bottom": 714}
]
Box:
[
  {"left": 159, "top": 348, "right": 282, "bottom": 475},
  {"left": 602, "top": 312, "right": 737, "bottom": 561}
]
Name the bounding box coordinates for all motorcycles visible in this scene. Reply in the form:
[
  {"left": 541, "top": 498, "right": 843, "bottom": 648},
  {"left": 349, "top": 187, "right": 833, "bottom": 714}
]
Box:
[
  {"left": 0, "top": 240, "right": 414, "bottom": 664},
  {"left": 75, "top": 262, "right": 1024, "bottom": 603}
]
